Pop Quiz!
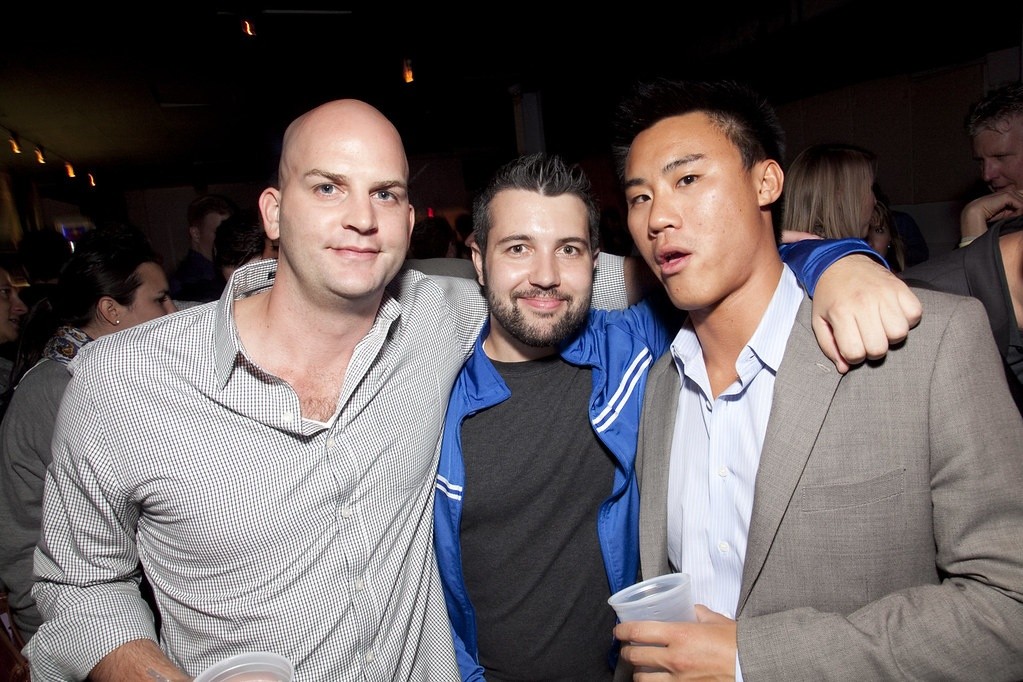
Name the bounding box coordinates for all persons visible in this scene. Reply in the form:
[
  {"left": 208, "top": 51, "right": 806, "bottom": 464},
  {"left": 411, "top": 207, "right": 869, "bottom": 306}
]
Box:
[
  {"left": 20, "top": 98, "right": 828, "bottom": 682},
  {"left": 433, "top": 152, "right": 924, "bottom": 682},
  {"left": 7, "top": 236, "right": 182, "bottom": 655},
  {"left": 1, "top": 91, "right": 1021, "bottom": 413},
  {"left": 604, "top": 80, "right": 1009, "bottom": 682}
]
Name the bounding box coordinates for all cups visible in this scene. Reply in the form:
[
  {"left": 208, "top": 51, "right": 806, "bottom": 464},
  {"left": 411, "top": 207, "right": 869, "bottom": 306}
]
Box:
[
  {"left": 608, "top": 571, "right": 701, "bottom": 675},
  {"left": 190, "top": 652, "right": 294, "bottom": 682}
]
